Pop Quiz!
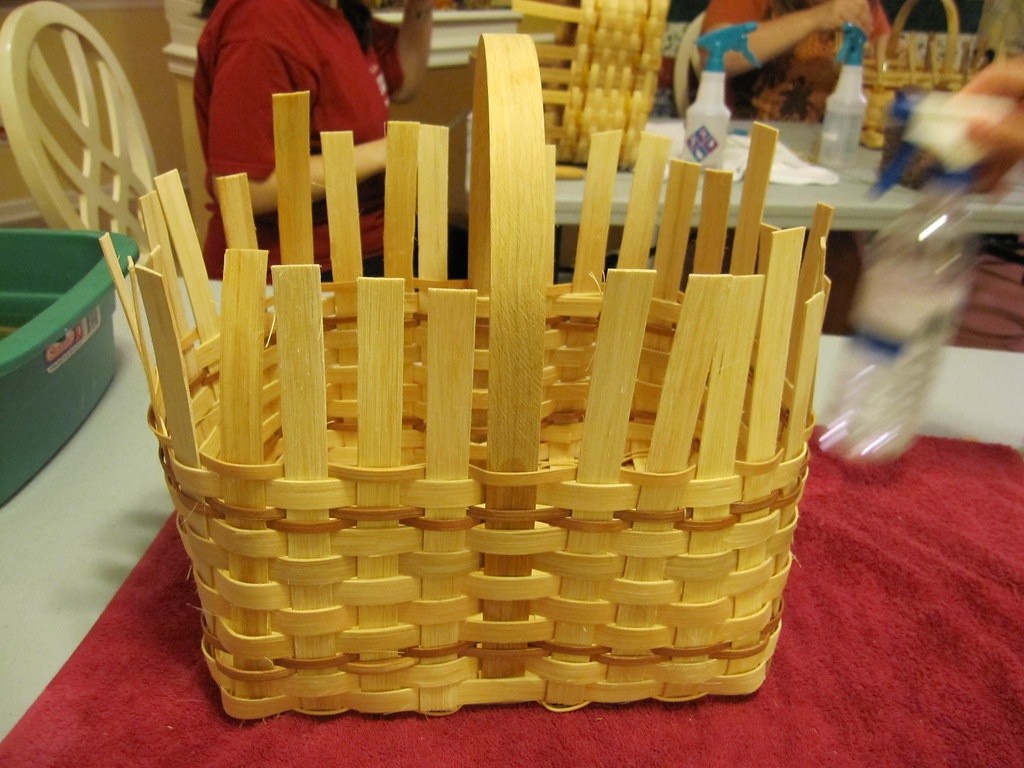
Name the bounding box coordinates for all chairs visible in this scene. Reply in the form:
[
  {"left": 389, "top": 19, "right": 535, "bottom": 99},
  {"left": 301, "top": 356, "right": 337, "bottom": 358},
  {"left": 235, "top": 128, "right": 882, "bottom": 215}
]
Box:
[{"left": 0, "top": 1, "right": 160, "bottom": 254}]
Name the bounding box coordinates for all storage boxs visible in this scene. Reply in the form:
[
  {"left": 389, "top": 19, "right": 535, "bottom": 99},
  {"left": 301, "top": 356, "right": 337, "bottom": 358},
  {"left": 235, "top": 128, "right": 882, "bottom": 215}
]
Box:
[{"left": 0, "top": 228, "right": 139, "bottom": 509}]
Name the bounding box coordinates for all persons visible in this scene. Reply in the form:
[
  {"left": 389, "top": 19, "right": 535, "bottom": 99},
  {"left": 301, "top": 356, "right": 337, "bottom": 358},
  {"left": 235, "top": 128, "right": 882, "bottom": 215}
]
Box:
[
  {"left": 698, "top": 1, "right": 893, "bottom": 120},
  {"left": 192, "top": 0, "right": 469, "bottom": 285},
  {"left": 953, "top": 59, "right": 1024, "bottom": 160}
]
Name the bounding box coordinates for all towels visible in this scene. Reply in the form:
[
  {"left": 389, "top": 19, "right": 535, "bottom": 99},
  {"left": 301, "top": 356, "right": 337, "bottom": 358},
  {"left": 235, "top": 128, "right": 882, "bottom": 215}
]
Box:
[
  {"left": 0, "top": 404, "right": 1023, "bottom": 768},
  {"left": 644, "top": 120, "right": 842, "bottom": 187}
]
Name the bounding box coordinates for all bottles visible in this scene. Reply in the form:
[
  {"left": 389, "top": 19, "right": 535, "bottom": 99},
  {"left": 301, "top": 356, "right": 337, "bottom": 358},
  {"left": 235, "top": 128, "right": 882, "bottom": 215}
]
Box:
[{"left": 820, "top": 176, "right": 968, "bottom": 464}]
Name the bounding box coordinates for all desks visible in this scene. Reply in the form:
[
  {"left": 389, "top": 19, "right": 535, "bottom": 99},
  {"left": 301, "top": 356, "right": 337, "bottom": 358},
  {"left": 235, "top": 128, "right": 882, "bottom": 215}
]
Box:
[{"left": 0, "top": 123, "right": 1024, "bottom": 768}]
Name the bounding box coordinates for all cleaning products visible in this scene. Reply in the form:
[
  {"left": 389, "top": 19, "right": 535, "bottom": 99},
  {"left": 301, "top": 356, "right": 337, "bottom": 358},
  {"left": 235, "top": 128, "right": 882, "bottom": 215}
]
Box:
[
  {"left": 679, "top": 20, "right": 764, "bottom": 172},
  {"left": 813, "top": 18, "right": 868, "bottom": 173}
]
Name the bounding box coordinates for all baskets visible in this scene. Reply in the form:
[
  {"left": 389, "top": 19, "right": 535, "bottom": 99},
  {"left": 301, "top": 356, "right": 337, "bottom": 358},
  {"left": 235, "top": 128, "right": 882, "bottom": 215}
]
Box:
[
  {"left": 857, "top": 0, "right": 980, "bottom": 151},
  {"left": 508, "top": 1, "right": 672, "bottom": 174},
  {"left": 99, "top": 32, "right": 835, "bottom": 722}
]
